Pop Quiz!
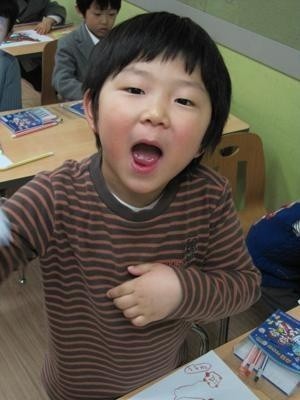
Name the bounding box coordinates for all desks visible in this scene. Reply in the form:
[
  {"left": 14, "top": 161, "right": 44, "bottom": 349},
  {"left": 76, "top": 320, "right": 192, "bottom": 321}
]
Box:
[
  {"left": 116, "top": 306, "right": 300, "bottom": 400},
  {"left": 0, "top": 20, "right": 77, "bottom": 52},
  {"left": 0, "top": 99, "right": 250, "bottom": 192}
]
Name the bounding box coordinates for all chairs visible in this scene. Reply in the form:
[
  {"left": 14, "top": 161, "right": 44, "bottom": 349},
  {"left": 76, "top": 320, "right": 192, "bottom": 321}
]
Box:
[
  {"left": 200, "top": 131, "right": 279, "bottom": 347},
  {"left": 40, "top": 40, "right": 67, "bottom": 105}
]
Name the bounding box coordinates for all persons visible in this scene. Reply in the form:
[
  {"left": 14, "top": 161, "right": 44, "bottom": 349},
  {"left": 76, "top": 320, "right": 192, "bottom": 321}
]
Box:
[
  {"left": 15, "top": 0, "right": 68, "bottom": 93},
  {"left": 0, "top": 12, "right": 266, "bottom": 399},
  {"left": 0, "top": 1, "right": 24, "bottom": 114},
  {"left": 50, "top": 0, "right": 121, "bottom": 103}
]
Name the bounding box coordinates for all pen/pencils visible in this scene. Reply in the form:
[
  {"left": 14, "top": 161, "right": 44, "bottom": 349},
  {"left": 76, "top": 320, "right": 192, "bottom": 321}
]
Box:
[
  {"left": 11, "top": 118, "right": 63, "bottom": 139},
  {"left": 239, "top": 344, "right": 269, "bottom": 382},
  {"left": 0, "top": 151, "right": 54, "bottom": 171}
]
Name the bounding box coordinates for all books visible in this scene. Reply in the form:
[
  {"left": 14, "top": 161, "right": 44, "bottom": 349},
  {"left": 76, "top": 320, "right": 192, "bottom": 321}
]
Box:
[
  {"left": 0, "top": 106, "right": 58, "bottom": 134},
  {"left": 65, "top": 101, "right": 86, "bottom": 117}
]
